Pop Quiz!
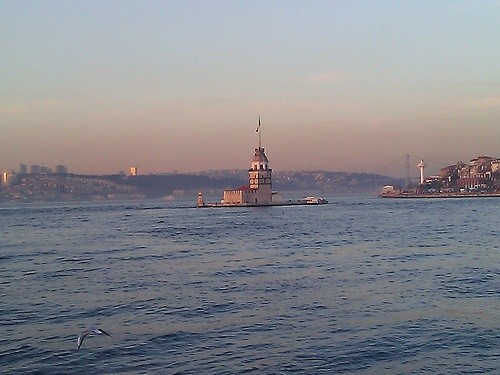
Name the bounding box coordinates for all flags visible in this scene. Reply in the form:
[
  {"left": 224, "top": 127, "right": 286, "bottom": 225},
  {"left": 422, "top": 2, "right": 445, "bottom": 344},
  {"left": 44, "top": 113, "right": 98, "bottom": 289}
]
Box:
[{"left": 255, "top": 118, "right": 260, "bottom": 133}]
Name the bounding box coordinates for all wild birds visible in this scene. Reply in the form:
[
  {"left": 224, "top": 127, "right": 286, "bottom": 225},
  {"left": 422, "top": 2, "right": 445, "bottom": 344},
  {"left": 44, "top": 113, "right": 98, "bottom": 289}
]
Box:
[{"left": 76, "top": 326, "right": 112, "bottom": 351}]
